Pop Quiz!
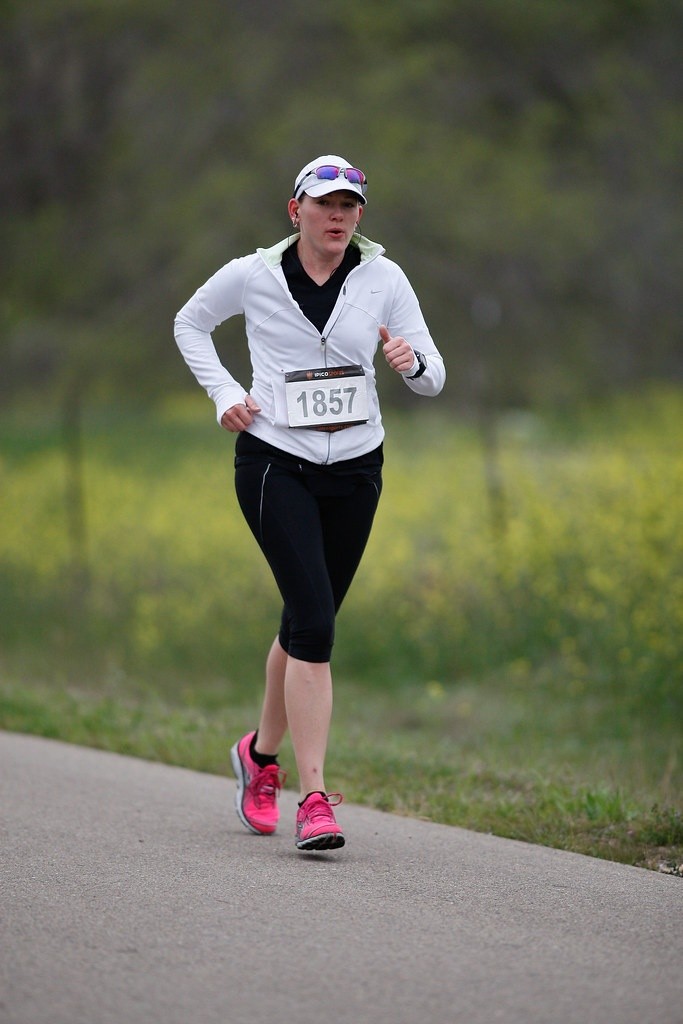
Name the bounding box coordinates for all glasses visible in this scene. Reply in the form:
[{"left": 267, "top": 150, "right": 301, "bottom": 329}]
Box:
[{"left": 292, "top": 165, "right": 370, "bottom": 198}]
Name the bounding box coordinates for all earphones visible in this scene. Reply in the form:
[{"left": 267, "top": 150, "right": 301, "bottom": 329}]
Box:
[{"left": 293, "top": 212, "right": 298, "bottom": 225}]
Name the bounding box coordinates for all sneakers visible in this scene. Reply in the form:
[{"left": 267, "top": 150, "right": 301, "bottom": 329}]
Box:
[
  {"left": 294, "top": 793, "right": 344, "bottom": 850},
  {"left": 228, "top": 729, "right": 288, "bottom": 835}
]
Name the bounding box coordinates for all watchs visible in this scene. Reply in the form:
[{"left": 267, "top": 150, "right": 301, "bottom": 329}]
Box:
[{"left": 405, "top": 349, "right": 427, "bottom": 381}]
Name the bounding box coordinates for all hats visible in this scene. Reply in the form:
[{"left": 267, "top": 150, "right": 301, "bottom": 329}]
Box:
[{"left": 293, "top": 154, "right": 367, "bottom": 206}]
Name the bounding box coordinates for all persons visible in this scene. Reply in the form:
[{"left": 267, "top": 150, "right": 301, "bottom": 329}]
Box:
[{"left": 174, "top": 154, "right": 447, "bottom": 851}]
